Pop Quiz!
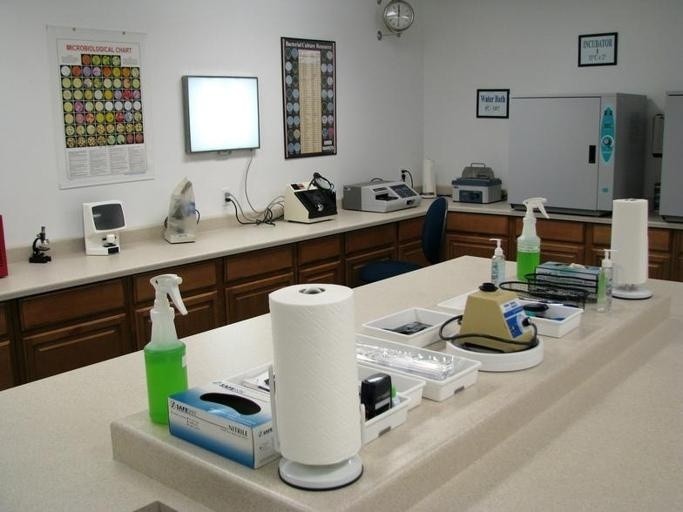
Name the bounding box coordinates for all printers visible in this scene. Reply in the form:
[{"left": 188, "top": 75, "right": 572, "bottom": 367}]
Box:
[{"left": 344, "top": 178, "right": 422, "bottom": 211}]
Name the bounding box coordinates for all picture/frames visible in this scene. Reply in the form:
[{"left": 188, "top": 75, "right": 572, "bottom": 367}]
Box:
[
  {"left": 476, "top": 88, "right": 509, "bottom": 118},
  {"left": 578, "top": 32, "right": 617, "bottom": 66},
  {"left": 281, "top": 36, "right": 337, "bottom": 158}
]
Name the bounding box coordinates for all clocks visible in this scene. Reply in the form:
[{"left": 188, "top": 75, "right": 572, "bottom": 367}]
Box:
[{"left": 383, "top": 2, "right": 415, "bottom": 30}]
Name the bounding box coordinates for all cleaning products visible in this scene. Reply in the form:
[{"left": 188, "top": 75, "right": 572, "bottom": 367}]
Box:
[
  {"left": 515, "top": 197, "right": 550, "bottom": 282},
  {"left": 141, "top": 272, "right": 189, "bottom": 427}
]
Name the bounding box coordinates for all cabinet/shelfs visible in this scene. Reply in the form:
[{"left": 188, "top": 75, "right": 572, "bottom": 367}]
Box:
[
  {"left": 133, "top": 243, "right": 298, "bottom": 350},
  {"left": 0, "top": 276, "right": 134, "bottom": 393},
  {"left": 397, "top": 216, "right": 431, "bottom": 267},
  {"left": 441, "top": 212, "right": 509, "bottom": 261},
  {"left": 298, "top": 223, "right": 398, "bottom": 288},
  {"left": 511, "top": 217, "right": 672, "bottom": 282}
]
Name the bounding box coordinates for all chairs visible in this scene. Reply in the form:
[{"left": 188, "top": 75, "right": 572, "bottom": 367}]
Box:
[{"left": 362, "top": 198, "right": 447, "bottom": 281}]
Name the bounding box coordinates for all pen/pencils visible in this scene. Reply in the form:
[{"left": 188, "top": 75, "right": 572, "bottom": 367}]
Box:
[{"left": 539, "top": 301, "right": 560, "bottom": 304}]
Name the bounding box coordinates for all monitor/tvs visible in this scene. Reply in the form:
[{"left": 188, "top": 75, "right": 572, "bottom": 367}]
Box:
[{"left": 181, "top": 75, "right": 263, "bottom": 154}]
